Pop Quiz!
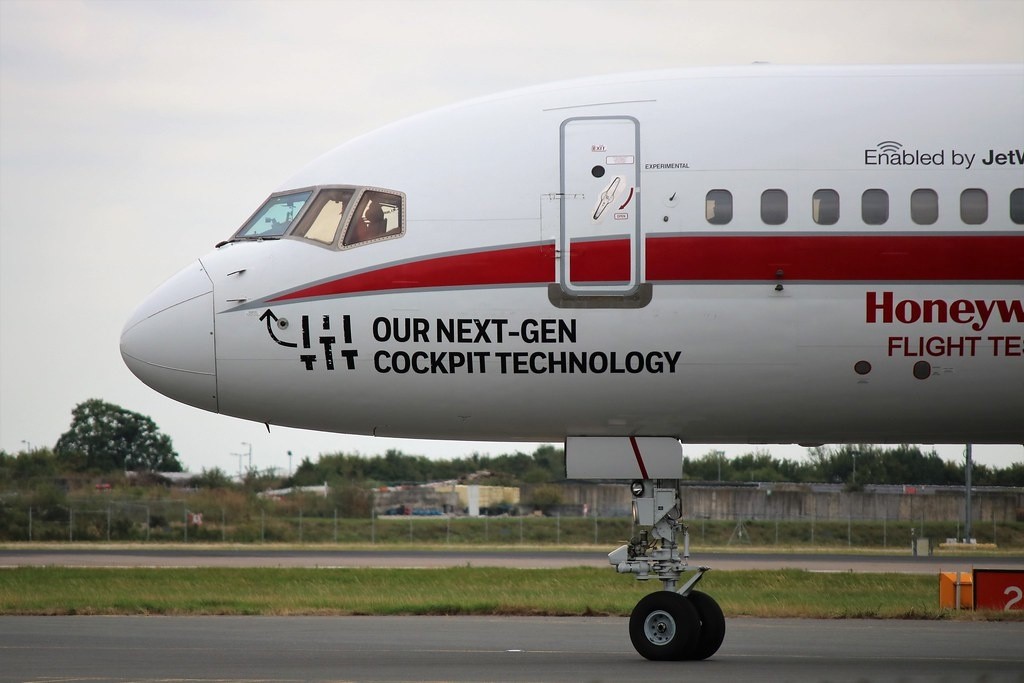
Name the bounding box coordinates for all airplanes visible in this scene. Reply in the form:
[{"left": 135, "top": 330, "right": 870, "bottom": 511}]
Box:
[{"left": 118, "top": 60, "right": 1022, "bottom": 661}]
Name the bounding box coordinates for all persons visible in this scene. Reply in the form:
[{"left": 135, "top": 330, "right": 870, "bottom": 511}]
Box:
[{"left": 340, "top": 202, "right": 371, "bottom": 246}]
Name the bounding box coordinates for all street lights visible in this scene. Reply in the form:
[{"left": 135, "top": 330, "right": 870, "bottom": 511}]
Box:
[
  {"left": 231, "top": 452, "right": 248, "bottom": 478},
  {"left": 241, "top": 441, "right": 251, "bottom": 470},
  {"left": 287, "top": 450, "right": 293, "bottom": 478}
]
